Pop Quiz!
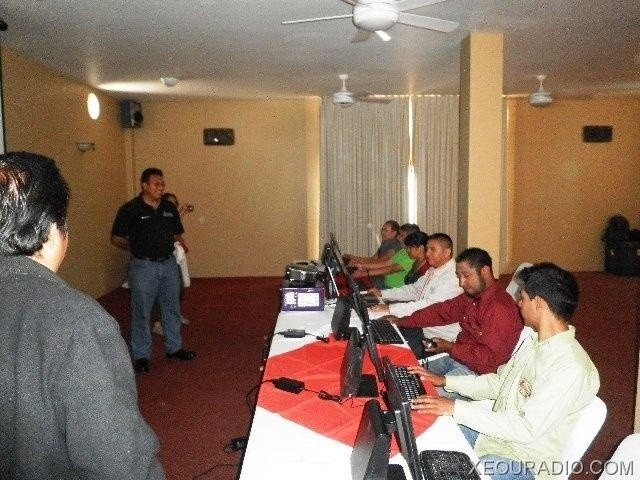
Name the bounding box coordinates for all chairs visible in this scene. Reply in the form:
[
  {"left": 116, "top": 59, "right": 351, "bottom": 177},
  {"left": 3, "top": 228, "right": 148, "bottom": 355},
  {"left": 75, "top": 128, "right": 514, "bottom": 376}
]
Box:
[
  {"left": 507, "top": 259, "right": 532, "bottom": 302},
  {"left": 563, "top": 394, "right": 607, "bottom": 480}
]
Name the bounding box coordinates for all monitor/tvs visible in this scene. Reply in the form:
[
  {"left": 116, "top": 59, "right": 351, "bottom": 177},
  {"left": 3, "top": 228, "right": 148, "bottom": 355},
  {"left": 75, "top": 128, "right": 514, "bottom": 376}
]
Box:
[
  {"left": 321, "top": 235, "right": 361, "bottom": 297},
  {"left": 350, "top": 356, "right": 423, "bottom": 480},
  {"left": 331, "top": 272, "right": 370, "bottom": 340},
  {"left": 340, "top": 320, "right": 385, "bottom": 397}
]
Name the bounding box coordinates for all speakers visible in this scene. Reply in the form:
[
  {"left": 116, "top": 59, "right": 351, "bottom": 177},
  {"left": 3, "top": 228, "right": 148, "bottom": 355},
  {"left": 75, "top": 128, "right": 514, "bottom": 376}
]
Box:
[{"left": 121, "top": 100, "right": 144, "bottom": 128}]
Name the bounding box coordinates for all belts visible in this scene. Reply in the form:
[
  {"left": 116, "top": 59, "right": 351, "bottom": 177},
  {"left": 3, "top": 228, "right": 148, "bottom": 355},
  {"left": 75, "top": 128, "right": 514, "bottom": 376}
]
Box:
[{"left": 131, "top": 248, "right": 176, "bottom": 262}]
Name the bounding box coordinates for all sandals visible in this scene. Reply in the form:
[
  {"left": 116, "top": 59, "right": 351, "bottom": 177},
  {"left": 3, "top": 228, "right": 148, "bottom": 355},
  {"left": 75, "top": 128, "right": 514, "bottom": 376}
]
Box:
[
  {"left": 178, "top": 311, "right": 188, "bottom": 323},
  {"left": 148, "top": 320, "right": 165, "bottom": 337}
]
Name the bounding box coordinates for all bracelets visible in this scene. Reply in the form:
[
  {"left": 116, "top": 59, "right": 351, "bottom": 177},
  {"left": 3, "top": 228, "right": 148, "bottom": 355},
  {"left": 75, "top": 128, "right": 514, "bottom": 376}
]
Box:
[{"left": 367, "top": 270, "right": 369, "bottom": 277}]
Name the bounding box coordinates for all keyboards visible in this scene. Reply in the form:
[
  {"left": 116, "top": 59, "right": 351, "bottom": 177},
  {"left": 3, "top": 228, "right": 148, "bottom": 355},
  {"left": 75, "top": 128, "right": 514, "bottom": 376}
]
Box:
[
  {"left": 420, "top": 450, "right": 480, "bottom": 480},
  {"left": 371, "top": 319, "right": 404, "bottom": 344},
  {"left": 362, "top": 291, "right": 380, "bottom": 308},
  {"left": 392, "top": 365, "right": 427, "bottom": 411}
]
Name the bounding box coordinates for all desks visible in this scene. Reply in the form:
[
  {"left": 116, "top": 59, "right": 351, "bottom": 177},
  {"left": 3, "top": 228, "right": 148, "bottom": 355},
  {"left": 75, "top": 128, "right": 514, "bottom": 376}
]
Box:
[{"left": 236, "top": 275, "right": 494, "bottom": 480}]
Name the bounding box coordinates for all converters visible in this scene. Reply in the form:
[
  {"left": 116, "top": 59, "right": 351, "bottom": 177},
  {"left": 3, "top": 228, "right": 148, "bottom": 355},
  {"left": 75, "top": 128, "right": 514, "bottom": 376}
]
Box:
[
  {"left": 283, "top": 328, "right": 306, "bottom": 339},
  {"left": 229, "top": 437, "right": 247, "bottom": 451},
  {"left": 271, "top": 374, "right": 306, "bottom": 395}
]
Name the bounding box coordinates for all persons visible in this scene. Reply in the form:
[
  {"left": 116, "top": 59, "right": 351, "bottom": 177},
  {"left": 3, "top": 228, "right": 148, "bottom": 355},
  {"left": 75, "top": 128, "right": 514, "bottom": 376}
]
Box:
[
  {"left": 111, "top": 169, "right": 197, "bottom": 374},
  {"left": 378, "top": 247, "right": 525, "bottom": 400},
  {"left": 151, "top": 191, "right": 192, "bottom": 337},
  {"left": 342, "top": 220, "right": 399, "bottom": 263},
  {"left": 0, "top": 153, "right": 166, "bottom": 480},
  {"left": 367, "top": 232, "right": 466, "bottom": 319},
  {"left": 350, "top": 223, "right": 420, "bottom": 270},
  {"left": 405, "top": 261, "right": 601, "bottom": 480},
  {"left": 402, "top": 230, "right": 429, "bottom": 284}
]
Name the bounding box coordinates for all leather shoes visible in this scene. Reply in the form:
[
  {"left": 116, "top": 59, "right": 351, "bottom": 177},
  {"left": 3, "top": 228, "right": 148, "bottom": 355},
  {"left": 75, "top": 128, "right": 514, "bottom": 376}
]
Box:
[
  {"left": 131, "top": 356, "right": 152, "bottom": 376},
  {"left": 165, "top": 346, "right": 197, "bottom": 361}
]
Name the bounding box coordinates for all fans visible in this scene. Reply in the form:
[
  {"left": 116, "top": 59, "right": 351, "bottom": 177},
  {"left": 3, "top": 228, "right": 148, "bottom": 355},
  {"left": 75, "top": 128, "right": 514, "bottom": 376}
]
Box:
[
  {"left": 318, "top": 74, "right": 391, "bottom": 106},
  {"left": 505, "top": 57, "right": 596, "bottom": 107},
  {"left": 280, "top": 1, "right": 459, "bottom": 43}
]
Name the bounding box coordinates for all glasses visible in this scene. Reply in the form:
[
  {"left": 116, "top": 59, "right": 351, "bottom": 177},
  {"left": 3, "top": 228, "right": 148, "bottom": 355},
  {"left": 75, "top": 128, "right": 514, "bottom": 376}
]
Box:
[{"left": 380, "top": 228, "right": 392, "bottom": 232}]
